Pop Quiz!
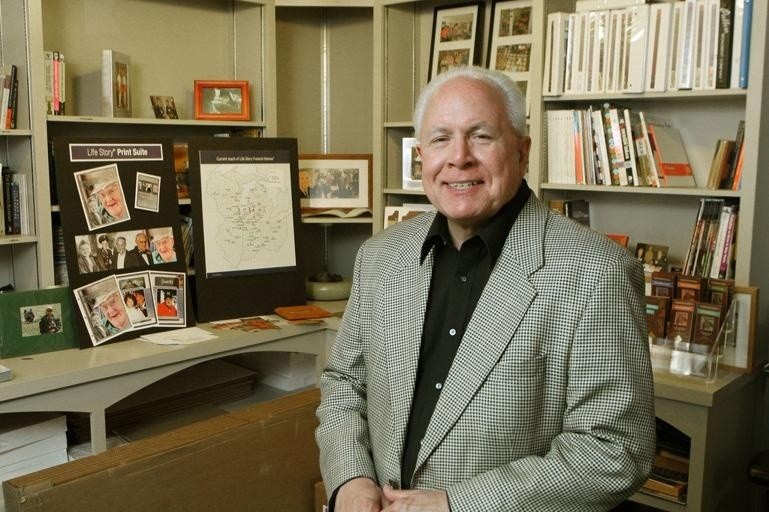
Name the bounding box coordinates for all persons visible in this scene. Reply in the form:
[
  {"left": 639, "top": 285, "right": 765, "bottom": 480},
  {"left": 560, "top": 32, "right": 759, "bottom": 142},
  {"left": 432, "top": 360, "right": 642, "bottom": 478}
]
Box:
[
  {"left": 299, "top": 169, "right": 359, "bottom": 199},
  {"left": 637, "top": 246, "right": 666, "bottom": 266},
  {"left": 86, "top": 197, "right": 106, "bottom": 229},
  {"left": 156, "top": 97, "right": 166, "bottom": 118},
  {"left": 437, "top": 13, "right": 531, "bottom": 72},
  {"left": 85, "top": 278, "right": 177, "bottom": 341},
  {"left": 117, "top": 68, "right": 127, "bottom": 108},
  {"left": 77, "top": 229, "right": 177, "bottom": 274},
  {"left": 315, "top": 64, "right": 658, "bottom": 512},
  {"left": 165, "top": 100, "right": 177, "bottom": 119},
  {"left": 88, "top": 168, "right": 129, "bottom": 225}
]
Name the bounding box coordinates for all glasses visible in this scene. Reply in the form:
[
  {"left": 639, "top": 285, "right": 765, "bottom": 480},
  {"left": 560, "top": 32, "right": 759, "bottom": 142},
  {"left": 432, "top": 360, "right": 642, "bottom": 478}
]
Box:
[
  {"left": 138, "top": 240, "right": 147, "bottom": 243},
  {"left": 97, "top": 189, "right": 117, "bottom": 199},
  {"left": 101, "top": 297, "right": 120, "bottom": 311},
  {"left": 156, "top": 238, "right": 172, "bottom": 246},
  {"left": 47, "top": 310, "right": 52, "bottom": 312}
]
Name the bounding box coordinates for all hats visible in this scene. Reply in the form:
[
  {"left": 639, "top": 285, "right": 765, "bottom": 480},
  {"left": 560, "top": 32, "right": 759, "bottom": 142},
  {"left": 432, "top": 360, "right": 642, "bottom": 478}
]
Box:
[
  {"left": 151, "top": 228, "right": 173, "bottom": 243},
  {"left": 46, "top": 306, "right": 53, "bottom": 311},
  {"left": 99, "top": 235, "right": 109, "bottom": 243},
  {"left": 91, "top": 174, "right": 117, "bottom": 195},
  {"left": 91, "top": 280, "right": 118, "bottom": 309}
]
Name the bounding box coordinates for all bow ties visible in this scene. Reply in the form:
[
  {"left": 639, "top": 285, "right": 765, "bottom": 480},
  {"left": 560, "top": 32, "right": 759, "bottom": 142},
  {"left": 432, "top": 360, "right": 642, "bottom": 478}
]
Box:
[{"left": 140, "top": 250, "right": 147, "bottom": 255}]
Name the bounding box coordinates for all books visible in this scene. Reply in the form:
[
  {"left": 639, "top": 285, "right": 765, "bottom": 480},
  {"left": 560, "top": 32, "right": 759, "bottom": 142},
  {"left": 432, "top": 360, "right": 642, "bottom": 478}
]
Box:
[
  {"left": 706, "top": 119, "right": 745, "bottom": 191},
  {"left": 551, "top": 199, "right": 590, "bottom": 227},
  {"left": 637, "top": 431, "right": 689, "bottom": 506},
  {"left": 0, "top": 162, "right": 29, "bottom": 235},
  {"left": 0, "top": 65, "right": 19, "bottom": 129},
  {"left": 540, "top": 1, "right": 752, "bottom": 94},
  {"left": 542, "top": 108, "right": 697, "bottom": 188},
  {"left": 44, "top": 51, "right": 65, "bottom": 115},
  {"left": 682, "top": 197, "right": 736, "bottom": 278}
]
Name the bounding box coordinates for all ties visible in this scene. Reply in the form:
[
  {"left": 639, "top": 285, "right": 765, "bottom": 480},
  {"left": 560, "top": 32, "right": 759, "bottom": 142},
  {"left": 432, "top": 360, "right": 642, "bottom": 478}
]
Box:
[{"left": 304, "top": 191, "right": 308, "bottom": 198}]
